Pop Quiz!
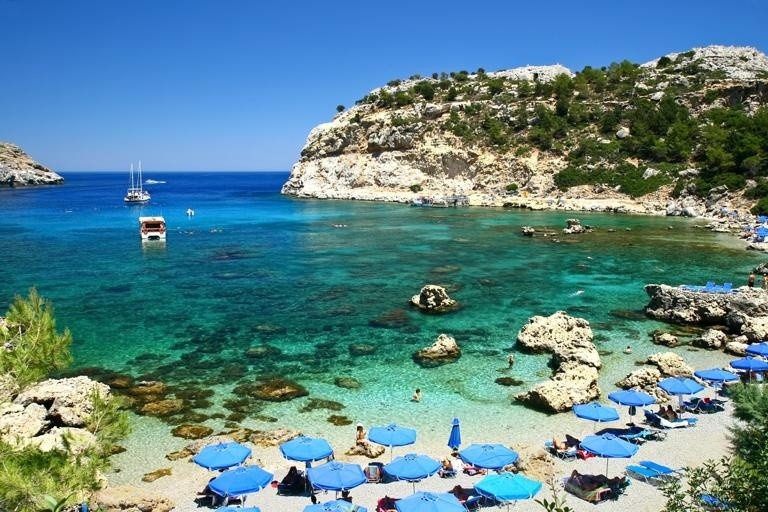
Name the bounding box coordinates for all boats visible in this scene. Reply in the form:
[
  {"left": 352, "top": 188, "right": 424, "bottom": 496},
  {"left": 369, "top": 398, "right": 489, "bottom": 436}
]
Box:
[
  {"left": 137, "top": 214, "right": 168, "bottom": 242},
  {"left": 145, "top": 179, "right": 167, "bottom": 184},
  {"left": 140, "top": 240, "right": 168, "bottom": 251}
]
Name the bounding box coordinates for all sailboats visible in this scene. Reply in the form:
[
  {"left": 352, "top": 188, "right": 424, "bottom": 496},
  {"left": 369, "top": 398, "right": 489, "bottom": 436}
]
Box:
[{"left": 123, "top": 159, "right": 151, "bottom": 205}]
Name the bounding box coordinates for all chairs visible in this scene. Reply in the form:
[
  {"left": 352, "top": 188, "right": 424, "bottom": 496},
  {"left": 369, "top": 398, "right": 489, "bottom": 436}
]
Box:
[
  {"left": 463, "top": 495, "right": 482, "bottom": 511},
  {"left": 439, "top": 465, "right": 455, "bottom": 478},
  {"left": 546, "top": 397, "right": 739, "bottom": 511},
  {"left": 681, "top": 281, "right": 734, "bottom": 294},
  {"left": 365, "top": 465, "right": 380, "bottom": 483}
]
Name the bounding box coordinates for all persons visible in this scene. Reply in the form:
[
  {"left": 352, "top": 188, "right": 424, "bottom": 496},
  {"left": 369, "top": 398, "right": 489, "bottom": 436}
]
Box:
[
  {"left": 748, "top": 271, "right": 755, "bottom": 287},
  {"left": 732, "top": 210, "right": 738, "bottom": 219},
  {"left": 761, "top": 273, "right": 768, "bottom": 291},
  {"left": 506, "top": 353, "right": 514, "bottom": 370},
  {"left": 412, "top": 388, "right": 421, "bottom": 401},
  {"left": 719, "top": 208, "right": 724, "bottom": 218},
  {"left": 552, "top": 435, "right": 576, "bottom": 451}
]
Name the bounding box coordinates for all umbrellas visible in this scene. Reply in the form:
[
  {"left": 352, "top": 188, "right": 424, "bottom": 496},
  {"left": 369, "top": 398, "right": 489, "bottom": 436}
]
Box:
[
  {"left": 758, "top": 215, "right": 768, "bottom": 222},
  {"left": 756, "top": 225, "right": 768, "bottom": 239},
  {"left": 572, "top": 343, "right": 767, "bottom": 489}
]
